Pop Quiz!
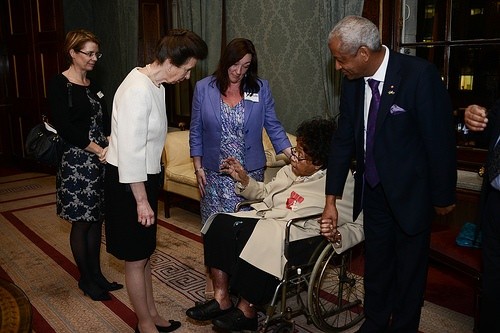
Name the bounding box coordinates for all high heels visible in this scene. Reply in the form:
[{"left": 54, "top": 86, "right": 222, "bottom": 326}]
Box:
[{"left": 78, "top": 271, "right": 124, "bottom": 301}]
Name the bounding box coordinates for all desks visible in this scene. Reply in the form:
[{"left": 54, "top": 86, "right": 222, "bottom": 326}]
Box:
[{"left": 0, "top": 277, "right": 33, "bottom": 333}]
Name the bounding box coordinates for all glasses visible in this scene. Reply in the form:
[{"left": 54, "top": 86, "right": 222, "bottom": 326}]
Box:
[
  {"left": 75, "top": 49, "right": 104, "bottom": 59},
  {"left": 291, "top": 147, "right": 306, "bottom": 162}
]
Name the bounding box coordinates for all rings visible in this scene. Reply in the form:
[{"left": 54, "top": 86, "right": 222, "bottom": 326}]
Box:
[{"left": 228, "top": 165, "right": 231, "bottom": 168}]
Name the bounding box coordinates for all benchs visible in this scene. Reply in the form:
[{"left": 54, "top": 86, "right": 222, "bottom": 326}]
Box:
[{"left": 162, "top": 127, "right": 298, "bottom": 220}]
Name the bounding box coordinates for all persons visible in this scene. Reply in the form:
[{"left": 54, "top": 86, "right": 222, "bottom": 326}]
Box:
[
  {"left": 321, "top": 15, "right": 458, "bottom": 333},
  {"left": 46, "top": 27, "right": 124, "bottom": 301},
  {"left": 465, "top": 68, "right": 500, "bottom": 333},
  {"left": 185, "top": 117, "right": 365, "bottom": 333},
  {"left": 188, "top": 38, "right": 294, "bottom": 302},
  {"left": 103, "top": 29, "right": 207, "bottom": 333}
]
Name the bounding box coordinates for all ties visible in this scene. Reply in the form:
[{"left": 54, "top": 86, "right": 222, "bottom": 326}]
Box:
[{"left": 365, "top": 79, "right": 380, "bottom": 188}]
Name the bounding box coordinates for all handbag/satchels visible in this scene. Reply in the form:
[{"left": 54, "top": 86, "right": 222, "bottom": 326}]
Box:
[
  {"left": 456, "top": 222, "right": 482, "bottom": 247},
  {"left": 24, "top": 75, "right": 72, "bottom": 176}
]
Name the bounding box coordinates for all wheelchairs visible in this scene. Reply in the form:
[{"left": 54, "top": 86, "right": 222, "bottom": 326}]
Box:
[{"left": 232, "top": 160, "right": 368, "bottom": 333}]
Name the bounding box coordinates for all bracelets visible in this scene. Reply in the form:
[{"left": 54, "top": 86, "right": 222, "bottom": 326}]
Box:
[
  {"left": 194, "top": 167, "right": 202, "bottom": 174},
  {"left": 335, "top": 233, "right": 341, "bottom": 245}
]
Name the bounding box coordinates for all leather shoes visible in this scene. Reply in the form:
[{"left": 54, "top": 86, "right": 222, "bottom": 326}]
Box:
[
  {"left": 135, "top": 320, "right": 181, "bottom": 333},
  {"left": 186, "top": 299, "right": 234, "bottom": 320},
  {"left": 213, "top": 308, "right": 258, "bottom": 331}
]
